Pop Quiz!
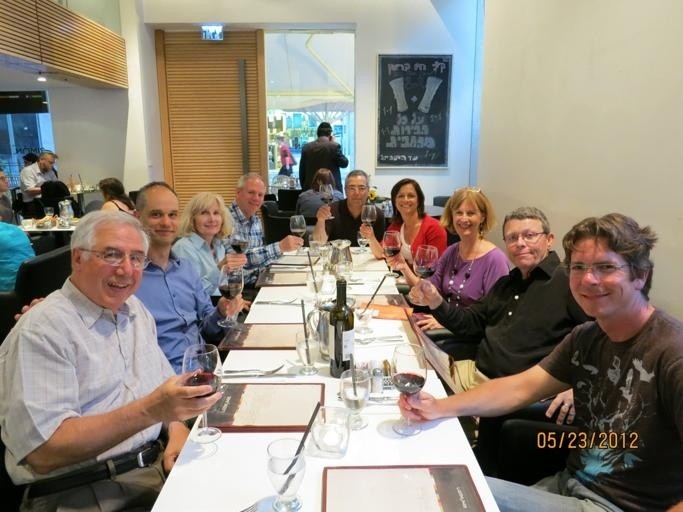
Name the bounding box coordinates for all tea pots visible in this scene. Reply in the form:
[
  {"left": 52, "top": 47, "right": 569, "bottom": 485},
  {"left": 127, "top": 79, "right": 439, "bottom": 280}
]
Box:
[
  {"left": 58, "top": 200, "right": 74, "bottom": 219},
  {"left": 329, "top": 240, "right": 353, "bottom": 276},
  {"left": 307, "top": 296, "right": 356, "bottom": 362}
]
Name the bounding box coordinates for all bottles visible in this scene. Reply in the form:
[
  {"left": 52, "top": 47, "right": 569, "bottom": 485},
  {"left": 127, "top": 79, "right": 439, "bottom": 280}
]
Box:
[{"left": 328, "top": 279, "right": 355, "bottom": 379}]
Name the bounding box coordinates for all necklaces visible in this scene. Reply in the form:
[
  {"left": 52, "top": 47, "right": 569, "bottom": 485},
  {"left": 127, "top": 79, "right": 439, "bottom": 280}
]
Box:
[{"left": 447, "top": 242, "right": 481, "bottom": 308}]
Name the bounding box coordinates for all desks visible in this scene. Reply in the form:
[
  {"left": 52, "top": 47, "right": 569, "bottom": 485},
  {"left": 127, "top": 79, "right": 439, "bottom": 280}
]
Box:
[{"left": 150, "top": 246, "right": 499, "bottom": 512}]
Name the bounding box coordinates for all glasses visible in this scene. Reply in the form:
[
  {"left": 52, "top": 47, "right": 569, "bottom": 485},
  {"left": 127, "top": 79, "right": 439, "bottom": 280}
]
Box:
[
  {"left": 502, "top": 231, "right": 546, "bottom": 242},
  {"left": 78, "top": 246, "right": 152, "bottom": 270},
  {"left": 451, "top": 187, "right": 481, "bottom": 192},
  {"left": 348, "top": 186, "right": 368, "bottom": 192},
  {"left": 568, "top": 262, "right": 632, "bottom": 275}
]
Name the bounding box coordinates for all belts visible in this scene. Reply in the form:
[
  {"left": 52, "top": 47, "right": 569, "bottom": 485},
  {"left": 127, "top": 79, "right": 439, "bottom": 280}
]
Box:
[{"left": 15, "top": 439, "right": 161, "bottom": 501}]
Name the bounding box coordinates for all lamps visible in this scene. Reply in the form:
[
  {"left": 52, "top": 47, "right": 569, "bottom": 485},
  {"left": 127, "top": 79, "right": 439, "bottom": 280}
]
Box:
[{"left": 36, "top": 71, "right": 47, "bottom": 83}]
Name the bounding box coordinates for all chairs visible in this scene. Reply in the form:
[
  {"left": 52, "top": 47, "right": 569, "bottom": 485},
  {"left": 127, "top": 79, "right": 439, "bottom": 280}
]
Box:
[
  {"left": 278, "top": 189, "right": 305, "bottom": 209},
  {"left": 13, "top": 244, "right": 71, "bottom": 306},
  {"left": 476, "top": 400, "right": 565, "bottom": 459},
  {"left": 492, "top": 419, "right": 576, "bottom": 481},
  {"left": 433, "top": 196, "right": 451, "bottom": 207},
  {"left": 261, "top": 201, "right": 295, "bottom": 243},
  {"left": 425, "top": 328, "right": 456, "bottom": 344},
  {"left": 396, "top": 282, "right": 413, "bottom": 295}
]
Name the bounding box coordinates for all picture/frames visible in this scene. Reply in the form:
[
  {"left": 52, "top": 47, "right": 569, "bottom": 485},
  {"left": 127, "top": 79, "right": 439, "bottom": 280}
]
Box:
[{"left": 375, "top": 52, "right": 454, "bottom": 170}]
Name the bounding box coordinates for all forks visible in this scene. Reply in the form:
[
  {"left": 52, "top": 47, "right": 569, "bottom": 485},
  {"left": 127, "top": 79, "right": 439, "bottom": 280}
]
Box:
[
  {"left": 224, "top": 364, "right": 285, "bottom": 377},
  {"left": 255, "top": 298, "right": 298, "bottom": 306}
]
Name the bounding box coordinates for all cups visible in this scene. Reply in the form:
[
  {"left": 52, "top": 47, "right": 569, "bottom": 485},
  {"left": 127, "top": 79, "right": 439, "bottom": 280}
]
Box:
[
  {"left": 355, "top": 297, "right": 374, "bottom": 344},
  {"left": 361, "top": 205, "right": 377, "bottom": 239},
  {"left": 230, "top": 236, "right": 250, "bottom": 254},
  {"left": 392, "top": 344, "right": 427, "bottom": 436},
  {"left": 217, "top": 264, "right": 244, "bottom": 328},
  {"left": 339, "top": 369, "right": 369, "bottom": 430},
  {"left": 44, "top": 207, "right": 54, "bottom": 217},
  {"left": 309, "top": 234, "right": 320, "bottom": 255},
  {"left": 296, "top": 331, "right": 320, "bottom": 377},
  {"left": 290, "top": 215, "right": 306, "bottom": 254},
  {"left": 319, "top": 241, "right": 329, "bottom": 264},
  {"left": 182, "top": 343, "right": 223, "bottom": 445},
  {"left": 309, "top": 405, "right": 351, "bottom": 460},
  {"left": 306, "top": 268, "right": 323, "bottom": 294},
  {"left": 383, "top": 231, "right": 401, "bottom": 278},
  {"left": 411, "top": 245, "right": 438, "bottom": 306},
  {"left": 357, "top": 232, "right": 368, "bottom": 254},
  {"left": 337, "top": 261, "right": 352, "bottom": 282},
  {"left": 267, "top": 437, "right": 307, "bottom": 512}
]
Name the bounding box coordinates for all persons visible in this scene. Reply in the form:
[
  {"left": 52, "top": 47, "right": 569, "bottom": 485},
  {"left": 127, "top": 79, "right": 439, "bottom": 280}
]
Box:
[
  {"left": 312, "top": 169, "right": 386, "bottom": 247},
  {"left": 20, "top": 150, "right": 59, "bottom": 217},
  {"left": 275, "top": 133, "right": 298, "bottom": 176},
  {"left": 381, "top": 185, "right": 511, "bottom": 331},
  {"left": 0, "top": 221, "right": 36, "bottom": 295},
  {"left": 295, "top": 166, "right": 345, "bottom": 246},
  {"left": 220, "top": 171, "right": 304, "bottom": 286},
  {"left": 171, "top": 191, "right": 251, "bottom": 313},
  {"left": 0, "top": 208, "right": 222, "bottom": 512},
  {"left": 408, "top": 206, "right": 597, "bottom": 425},
  {"left": 396, "top": 212, "right": 683, "bottom": 512},
  {"left": 359, "top": 178, "right": 448, "bottom": 273},
  {"left": 98, "top": 177, "right": 137, "bottom": 216},
  {"left": 298, "top": 121, "right": 349, "bottom": 192},
  {"left": 0, "top": 168, "right": 16, "bottom": 224},
  {"left": 132, "top": 182, "right": 246, "bottom": 384}
]
